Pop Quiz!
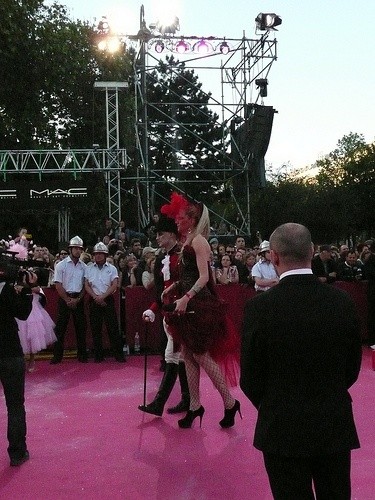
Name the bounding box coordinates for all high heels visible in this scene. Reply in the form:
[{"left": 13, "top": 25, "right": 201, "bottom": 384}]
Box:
[
  {"left": 219, "top": 399, "right": 242, "bottom": 428},
  {"left": 178, "top": 405, "right": 205, "bottom": 428}
]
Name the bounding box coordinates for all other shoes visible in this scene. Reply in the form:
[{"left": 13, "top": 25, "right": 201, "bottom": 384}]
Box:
[
  {"left": 77, "top": 355, "right": 88, "bottom": 363},
  {"left": 27, "top": 361, "right": 35, "bottom": 373},
  {"left": 115, "top": 352, "right": 125, "bottom": 362},
  {"left": 49, "top": 356, "right": 62, "bottom": 365},
  {"left": 10, "top": 450, "right": 29, "bottom": 466},
  {"left": 95, "top": 355, "right": 104, "bottom": 362}
]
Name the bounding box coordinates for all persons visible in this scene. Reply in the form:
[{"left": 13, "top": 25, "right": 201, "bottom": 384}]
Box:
[
  {"left": 139, "top": 217, "right": 190, "bottom": 415},
  {"left": 160, "top": 192, "right": 243, "bottom": 430},
  {"left": 84, "top": 242, "right": 126, "bottom": 362},
  {"left": 0, "top": 213, "right": 375, "bottom": 373},
  {"left": 0, "top": 271, "right": 37, "bottom": 467},
  {"left": 239, "top": 223, "right": 362, "bottom": 500},
  {"left": 48, "top": 236, "right": 88, "bottom": 364}
]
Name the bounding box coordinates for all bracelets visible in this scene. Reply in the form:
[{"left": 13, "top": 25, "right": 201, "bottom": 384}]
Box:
[
  {"left": 192, "top": 287, "right": 198, "bottom": 294},
  {"left": 186, "top": 293, "right": 191, "bottom": 300}
]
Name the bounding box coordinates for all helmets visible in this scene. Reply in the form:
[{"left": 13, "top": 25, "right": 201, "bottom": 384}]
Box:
[
  {"left": 259, "top": 239, "right": 270, "bottom": 253},
  {"left": 93, "top": 242, "right": 109, "bottom": 253},
  {"left": 69, "top": 236, "right": 83, "bottom": 248}
]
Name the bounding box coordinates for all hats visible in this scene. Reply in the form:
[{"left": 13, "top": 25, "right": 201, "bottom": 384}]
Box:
[
  {"left": 209, "top": 238, "right": 218, "bottom": 243},
  {"left": 154, "top": 213, "right": 181, "bottom": 237},
  {"left": 142, "top": 247, "right": 158, "bottom": 257},
  {"left": 320, "top": 245, "right": 334, "bottom": 253}
]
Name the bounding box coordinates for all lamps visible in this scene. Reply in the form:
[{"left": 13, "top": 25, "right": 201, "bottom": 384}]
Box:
[
  {"left": 149, "top": 15, "right": 181, "bottom": 37},
  {"left": 198, "top": 37, "right": 208, "bottom": 53},
  {"left": 175, "top": 37, "right": 190, "bottom": 54},
  {"left": 220, "top": 37, "right": 230, "bottom": 55},
  {"left": 255, "top": 11, "right": 282, "bottom": 36},
  {"left": 154, "top": 38, "right": 165, "bottom": 54}
]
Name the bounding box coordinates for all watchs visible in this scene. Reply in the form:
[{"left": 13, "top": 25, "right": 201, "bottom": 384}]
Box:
[{"left": 78, "top": 296, "right": 81, "bottom": 300}]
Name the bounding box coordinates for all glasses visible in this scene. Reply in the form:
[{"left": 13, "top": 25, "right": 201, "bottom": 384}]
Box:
[
  {"left": 117, "top": 252, "right": 123, "bottom": 255},
  {"left": 42, "top": 249, "right": 47, "bottom": 252},
  {"left": 252, "top": 248, "right": 259, "bottom": 250},
  {"left": 59, "top": 253, "right": 68, "bottom": 255},
  {"left": 133, "top": 244, "right": 140, "bottom": 246},
  {"left": 332, "top": 252, "right": 336, "bottom": 255},
  {"left": 36, "top": 250, "right": 42, "bottom": 251},
  {"left": 238, "top": 240, "right": 244, "bottom": 242},
  {"left": 225, "top": 251, "right": 235, "bottom": 254}
]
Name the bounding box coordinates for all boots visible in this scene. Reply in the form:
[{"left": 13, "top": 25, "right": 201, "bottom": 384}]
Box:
[
  {"left": 167, "top": 361, "right": 190, "bottom": 415},
  {"left": 138, "top": 363, "right": 180, "bottom": 416}
]
